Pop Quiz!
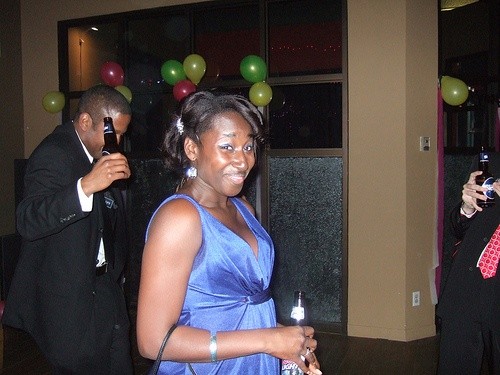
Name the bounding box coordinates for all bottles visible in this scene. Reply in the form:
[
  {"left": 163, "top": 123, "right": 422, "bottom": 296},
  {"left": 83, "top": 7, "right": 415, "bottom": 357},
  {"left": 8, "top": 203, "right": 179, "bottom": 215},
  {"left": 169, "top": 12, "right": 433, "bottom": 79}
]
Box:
[
  {"left": 475, "top": 146, "right": 495, "bottom": 208},
  {"left": 101, "top": 117, "right": 123, "bottom": 183},
  {"left": 280, "top": 291, "right": 309, "bottom": 375}
]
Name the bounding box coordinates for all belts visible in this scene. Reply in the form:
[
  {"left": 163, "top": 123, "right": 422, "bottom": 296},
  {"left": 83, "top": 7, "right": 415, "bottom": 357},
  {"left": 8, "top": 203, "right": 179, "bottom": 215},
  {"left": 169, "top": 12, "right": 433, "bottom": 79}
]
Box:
[{"left": 96, "top": 263, "right": 109, "bottom": 277}]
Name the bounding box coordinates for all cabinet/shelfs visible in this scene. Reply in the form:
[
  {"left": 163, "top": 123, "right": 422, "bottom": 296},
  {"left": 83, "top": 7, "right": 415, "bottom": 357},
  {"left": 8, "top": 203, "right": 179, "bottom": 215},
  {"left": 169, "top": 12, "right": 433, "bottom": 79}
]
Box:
[{"left": 446, "top": 47, "right": 500, "bottom": 155}]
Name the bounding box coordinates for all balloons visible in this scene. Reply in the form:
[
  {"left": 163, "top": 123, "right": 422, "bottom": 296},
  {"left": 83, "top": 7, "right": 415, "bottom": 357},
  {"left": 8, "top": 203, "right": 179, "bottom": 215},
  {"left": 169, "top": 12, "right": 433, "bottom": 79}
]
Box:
[
  {"left": 42, "top": 91, "right": 66, "bottom": 113},
  {"left": 240, "top": 55, "right": 267, "bottom": 83},
  {"left": 182, "top": 54, "right": 206, "bottom": 86},
  {"left": 161, "top": 60, "right": 186, "bottom": 86},
  {"left": 249, "top": 82, "right": 273, "bottom": 107},
  {"left": 98, "top": 60, "right": 125, "bottom": 88},
  {"left": 440, "top": 75, "right": 469, "bottom": 106},
  {"left": 115, "top": 85, "right": 132, "bottom": 103},
  {"left": 173, "top": 80, "right": 196, "bottom": 101}
]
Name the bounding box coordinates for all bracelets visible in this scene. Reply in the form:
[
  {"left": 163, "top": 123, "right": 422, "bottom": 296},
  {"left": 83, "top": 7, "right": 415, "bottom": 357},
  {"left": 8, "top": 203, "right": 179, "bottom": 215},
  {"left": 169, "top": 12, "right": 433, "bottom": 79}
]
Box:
[{"left": 210, "top": 330, "right": 217, "bottom": 362}]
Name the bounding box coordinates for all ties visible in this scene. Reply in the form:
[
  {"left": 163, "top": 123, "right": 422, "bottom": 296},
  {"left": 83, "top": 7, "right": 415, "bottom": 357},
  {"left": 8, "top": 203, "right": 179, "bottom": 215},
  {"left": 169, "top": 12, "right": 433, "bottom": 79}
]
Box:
[{"left": 479, "top": 224, "right": 500, "bottom": 279}]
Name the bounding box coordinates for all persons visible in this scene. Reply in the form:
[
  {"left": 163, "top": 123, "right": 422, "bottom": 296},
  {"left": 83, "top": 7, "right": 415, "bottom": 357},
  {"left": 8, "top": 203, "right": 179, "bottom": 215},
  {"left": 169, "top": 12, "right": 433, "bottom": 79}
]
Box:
[
  {"left": 136, "top": 91, "right": 323, "bottom": 375},
  {"left": 0, "top": 84, "right": 134, "bottom": 375},
  {"left": 437, "top": 155, "right": 500, "bottom": 375}
]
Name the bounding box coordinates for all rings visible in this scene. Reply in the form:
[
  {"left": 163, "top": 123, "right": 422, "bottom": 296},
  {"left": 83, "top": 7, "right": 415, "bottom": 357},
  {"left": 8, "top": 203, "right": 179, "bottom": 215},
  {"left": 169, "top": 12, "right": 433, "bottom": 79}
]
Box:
[{"left": 304, "top": 347, "right": 311, "bottom": 357}]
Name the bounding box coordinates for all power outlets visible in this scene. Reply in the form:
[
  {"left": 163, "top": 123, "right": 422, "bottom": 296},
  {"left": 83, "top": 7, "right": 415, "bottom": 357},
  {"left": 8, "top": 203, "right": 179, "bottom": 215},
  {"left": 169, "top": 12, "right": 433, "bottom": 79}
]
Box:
[{"left": 412, "top": 291, "right": 420, "bottom": 306}]
